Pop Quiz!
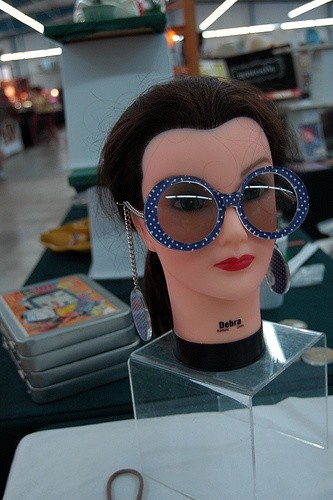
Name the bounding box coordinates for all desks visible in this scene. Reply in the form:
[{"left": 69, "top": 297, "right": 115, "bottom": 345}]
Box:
[{"left": 2, "top": 166, "right": 333, "bottom": 500}]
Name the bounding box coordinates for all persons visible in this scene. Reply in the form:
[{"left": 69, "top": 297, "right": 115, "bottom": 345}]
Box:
[{"left": 96, "top": 72, "right": 310, "bottom": 373}]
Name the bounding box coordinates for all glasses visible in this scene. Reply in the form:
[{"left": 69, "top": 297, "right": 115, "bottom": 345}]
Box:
[{"left": 127, "top": 166, "right": 309, "bottom": 252}]
